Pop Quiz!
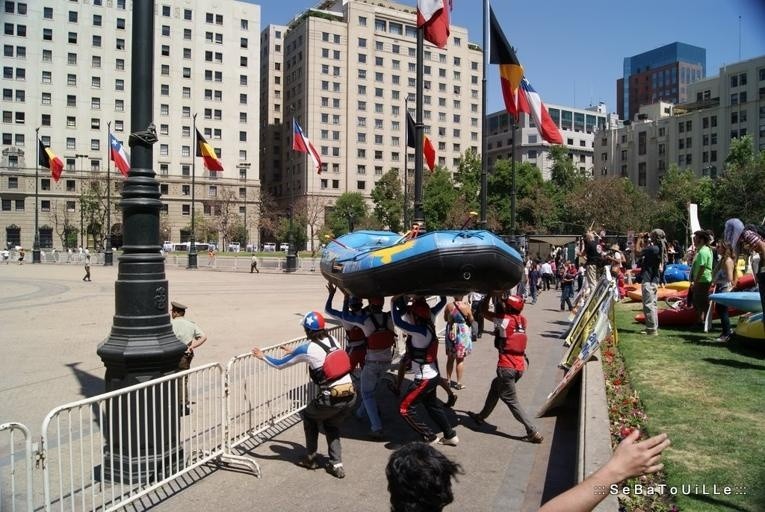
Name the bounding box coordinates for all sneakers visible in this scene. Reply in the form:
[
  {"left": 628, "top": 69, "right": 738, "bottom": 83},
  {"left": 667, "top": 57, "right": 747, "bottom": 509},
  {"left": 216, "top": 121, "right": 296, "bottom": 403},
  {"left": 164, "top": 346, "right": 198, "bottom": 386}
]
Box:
[
  {"left": 457, "top": 383, "right": 465, "bottom": 390},
  {"left": 295, "top": 453, "right": 318, "bottom": 469},
  {"left": 520, "top": 431, "right": 544, "bottom": 443},
  {"left": 443, "top": 393, "right": 457, "bottom": 408},
  {"left": 468, "top": 411, "right": 484, "bottom": 425},
  {"left": 388, "top": 381, "right": 400, "bottom": 396},
  {"left": 426, "top": 436, "right": 440, "bottom": 446},
  {"left": 640, "top": 330, "right": 657, "bottom": 336},
  {"left": 437, "top": 434, "right": 459, "bottom": 445},
  {"left": 367, "top": 425, "right": 387, "bottom": 439},
  {"left": 325, "top": 462, "right": 345, "bottom": 478},
  {"left": 716, "top": 328, "right": 733, "bottom": 342}
]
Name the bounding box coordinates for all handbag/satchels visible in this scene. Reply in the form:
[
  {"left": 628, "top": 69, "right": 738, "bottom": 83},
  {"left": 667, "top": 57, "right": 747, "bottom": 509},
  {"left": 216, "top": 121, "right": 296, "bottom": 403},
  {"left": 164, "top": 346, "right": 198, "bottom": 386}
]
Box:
[
  {"left": 612, "top": 266, "right": 619, "bottom": 272},
  {"left": 687, "top": 284, "right": 693, "bottom": 307}
]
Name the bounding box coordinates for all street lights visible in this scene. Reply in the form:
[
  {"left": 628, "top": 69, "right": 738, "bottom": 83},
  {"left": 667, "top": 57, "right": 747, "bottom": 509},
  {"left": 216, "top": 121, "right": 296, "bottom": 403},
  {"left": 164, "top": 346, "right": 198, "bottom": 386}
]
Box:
[
  {"left": 75, "top": 154, "right": 88, "bottom": 250},
  {"left": 238, "top": 162, "right": 251, "bottom": 252}
]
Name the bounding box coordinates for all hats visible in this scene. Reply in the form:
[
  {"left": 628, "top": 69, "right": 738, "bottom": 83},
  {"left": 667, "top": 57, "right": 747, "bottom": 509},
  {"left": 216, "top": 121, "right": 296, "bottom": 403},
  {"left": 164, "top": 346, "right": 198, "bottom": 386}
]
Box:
[
  {"left": 609, "top": 244, "right": 621, "bottom": 252},
  {"left": 171, "top": 302, "right": 187, "bottom": 312}
]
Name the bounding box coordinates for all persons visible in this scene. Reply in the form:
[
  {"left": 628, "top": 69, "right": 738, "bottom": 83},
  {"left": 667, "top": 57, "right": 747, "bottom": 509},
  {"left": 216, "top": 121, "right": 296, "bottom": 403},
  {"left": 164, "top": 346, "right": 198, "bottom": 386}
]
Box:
[
  {"left": 383, "top": 426, "right": 673, "bottom": 512},
  {"left": 169, "top": 301, "right": 207, "bottom": 417},
  {"left": 207, "top": 249, "right": 216, "bottom": 266},
  {"left": 0, "top": 244, "right": 102, "bottom": 265},
  {"left": 245, "top": 215, "right": 764, "bottom": 482},
  {"left": 82, "top": 255, "right": 92, "bottom": 282}
]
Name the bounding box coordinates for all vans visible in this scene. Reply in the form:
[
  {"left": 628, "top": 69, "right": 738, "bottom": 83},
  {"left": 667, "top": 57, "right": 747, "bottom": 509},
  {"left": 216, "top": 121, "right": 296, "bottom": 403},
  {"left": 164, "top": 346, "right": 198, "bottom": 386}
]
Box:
[{"left": 163, "top": 240, "right": 289, "bottom": 252}]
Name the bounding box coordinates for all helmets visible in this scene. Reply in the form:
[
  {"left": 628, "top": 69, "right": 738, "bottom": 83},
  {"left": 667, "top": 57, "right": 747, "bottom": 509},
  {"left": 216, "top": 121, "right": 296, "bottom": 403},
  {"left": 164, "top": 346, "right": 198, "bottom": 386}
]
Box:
[
  {"left": 348, "top": 294, "right": 362, "bottom": 306},
  {"left": 370, "top": 294, "right": 384, "bottom": 306},
  {"left": 298, "top": 311, "right": 324, "bottom": 331},
  {"left": 408, "top": 295, "right": 431, "bottom": 320},
  {"left": 505, "top": 294, "right": 524, "bottom": 312}
]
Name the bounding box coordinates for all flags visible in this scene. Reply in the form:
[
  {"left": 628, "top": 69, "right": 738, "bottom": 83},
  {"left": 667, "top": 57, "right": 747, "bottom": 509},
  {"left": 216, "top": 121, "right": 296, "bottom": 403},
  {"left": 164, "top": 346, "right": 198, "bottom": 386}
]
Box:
[
  {"left": 107, "top": 124, "right": 134, "bottom": 178},
  {"left": 511, "top": 49, "right": 565, "bottom": 147},
  {"left": 415, "top": 0, "right": 455, "bottom": 53},
  {"left": 483, "top": 0, "right": 522, "bottom": 122},
  {"left": 405, "top": 107, "right": 437, "bottom": 175},
  {"left": 291, "top": 116, "right": 324, "bottom": 176},
  {"left": 37, "top": 133, "right": 63, "bottom": 184},
  {"left": 194, "top": 126, "right": 225, "bottom": 174}
]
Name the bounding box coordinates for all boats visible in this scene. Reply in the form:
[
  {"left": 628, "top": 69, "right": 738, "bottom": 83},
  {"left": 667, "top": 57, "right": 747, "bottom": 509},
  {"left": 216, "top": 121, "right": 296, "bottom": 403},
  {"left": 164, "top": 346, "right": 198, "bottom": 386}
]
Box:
[
  {"left": 320, "top": 229, "right": 524, "bottom": 295},
  {"left": 626, "top": 254, "right": 765, "bottom": 345}
]
